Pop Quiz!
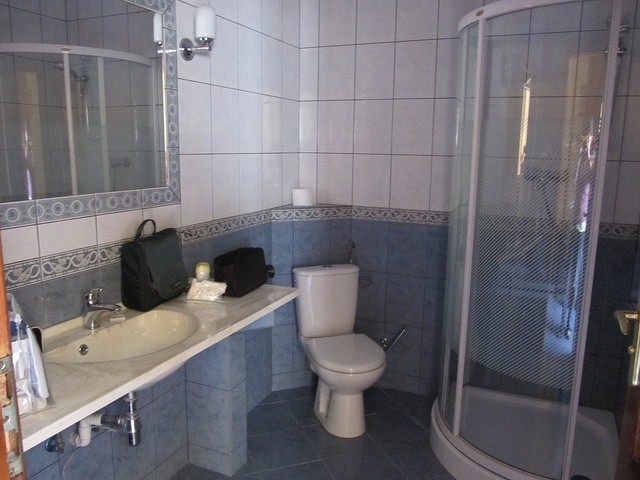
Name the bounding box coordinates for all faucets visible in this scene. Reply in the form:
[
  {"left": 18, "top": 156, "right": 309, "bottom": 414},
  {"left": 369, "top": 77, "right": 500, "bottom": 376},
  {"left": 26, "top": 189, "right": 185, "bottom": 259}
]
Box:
[{"left": 83, "top": 288, "right": 122, "bottom": 329}]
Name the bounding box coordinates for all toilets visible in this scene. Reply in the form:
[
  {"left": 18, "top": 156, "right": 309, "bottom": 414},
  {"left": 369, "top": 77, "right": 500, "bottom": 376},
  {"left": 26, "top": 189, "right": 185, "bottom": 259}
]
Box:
[{"left": 292, "top": 263, "right": 387, "bottom": 438}]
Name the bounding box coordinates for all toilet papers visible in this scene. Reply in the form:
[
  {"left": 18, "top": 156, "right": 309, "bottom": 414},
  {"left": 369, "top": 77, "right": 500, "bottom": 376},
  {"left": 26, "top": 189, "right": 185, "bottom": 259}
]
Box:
[{"left": 292, "top": 188, "right": 314, "bottom": 207}]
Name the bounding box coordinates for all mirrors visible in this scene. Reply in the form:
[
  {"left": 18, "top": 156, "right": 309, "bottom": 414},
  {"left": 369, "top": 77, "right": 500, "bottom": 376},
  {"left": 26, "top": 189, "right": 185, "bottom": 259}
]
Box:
[{"left": 0, "top": 0, "right": 181, "bottom": 230}]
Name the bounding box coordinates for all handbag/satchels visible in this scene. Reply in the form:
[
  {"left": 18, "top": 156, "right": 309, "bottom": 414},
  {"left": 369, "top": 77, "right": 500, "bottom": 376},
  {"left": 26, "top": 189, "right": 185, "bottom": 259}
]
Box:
[
  {"left": 213, "top": 247, "right": 275, "bottom": 298},
  {"left": 121, "top": 219, "right": 189, "bottom": 312}
]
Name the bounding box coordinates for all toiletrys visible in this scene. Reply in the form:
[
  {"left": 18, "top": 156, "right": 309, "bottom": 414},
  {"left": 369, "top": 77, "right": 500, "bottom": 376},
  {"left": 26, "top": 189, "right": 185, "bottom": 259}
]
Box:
[{"left": 9, "top": 320, "right": 50, "bottom": 417}]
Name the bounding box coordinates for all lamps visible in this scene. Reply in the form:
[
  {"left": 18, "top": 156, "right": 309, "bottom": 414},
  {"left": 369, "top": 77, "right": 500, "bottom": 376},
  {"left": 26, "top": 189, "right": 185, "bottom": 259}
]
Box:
[
  {"left": 190, "top": 3, "right": 216, "bottom": 55},
  {"left": 152, "top": 12, "right": 176, "bottom": 54}
]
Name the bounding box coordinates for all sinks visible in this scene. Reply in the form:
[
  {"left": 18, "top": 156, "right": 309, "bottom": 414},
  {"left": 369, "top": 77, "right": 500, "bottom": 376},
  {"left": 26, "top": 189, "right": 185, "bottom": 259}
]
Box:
[{"left": 42, "top": 308, "right": 200, "bottom": 365}]
